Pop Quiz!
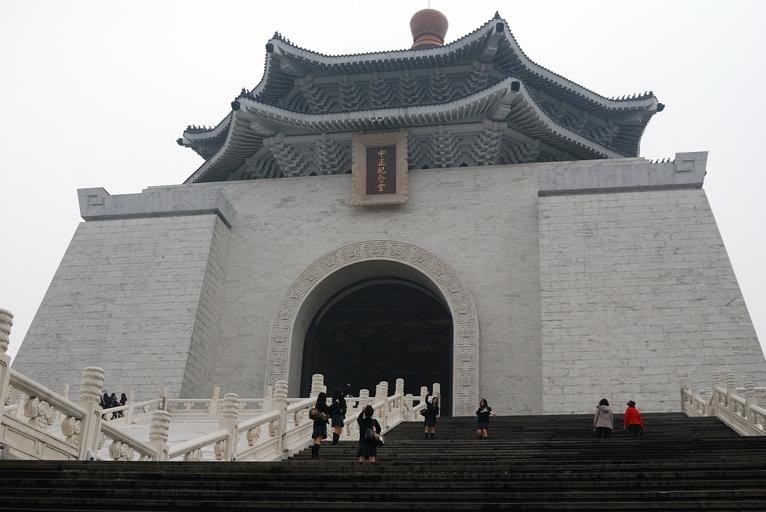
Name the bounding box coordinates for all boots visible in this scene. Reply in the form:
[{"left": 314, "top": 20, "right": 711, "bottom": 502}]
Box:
[
  {"left": 312, "top": 444, "right": 323, "bottom": 460},
  {"left": 424, "top": 433, "right": 435, "bottom": 440},
  {"left": 332, "top": 432, "right": 340, "bottom": 446}
]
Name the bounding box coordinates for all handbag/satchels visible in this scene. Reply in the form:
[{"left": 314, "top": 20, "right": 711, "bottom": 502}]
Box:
[
  {"left": 365, "top": 429, "right": 385, "bottom": 446},
  {"left": 309, "top": 409, "right": 328, "bottom": 422},
  {"left": 420, "top": 409, "right": 428, "bottom": 416},
  {"left": 327, "top": 404, "right": 339, "bottom": 416}
]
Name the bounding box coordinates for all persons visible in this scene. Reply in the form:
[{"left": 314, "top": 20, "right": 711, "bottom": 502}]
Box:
[
  {"left": 99, "top": 392, "right": 127, "bottom": 421},
  {"left": 356, "top": 405, "right": 382, "bottom": 462},
  {"left": 311, "top": 391, "right": 339, "bottom": 459},
  {"left": 423, "top": 392, "right": 439, "bottom": 439},
  {"left": 592, "top": 398, "right": 614, "bottom": 438},
  {"left": 623, "top": 400, "right": 644, "bottom": 438},
  {"left": 475, "top": 398, "right": 496, "bottom": 440},
  {"left": 331, "top": 383, "right": 351, "bottom": 446}
]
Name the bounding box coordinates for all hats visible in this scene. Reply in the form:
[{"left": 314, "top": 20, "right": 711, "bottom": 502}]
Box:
[{"left": 627, "top": 401, "right": 635, "bottom": 408}]
